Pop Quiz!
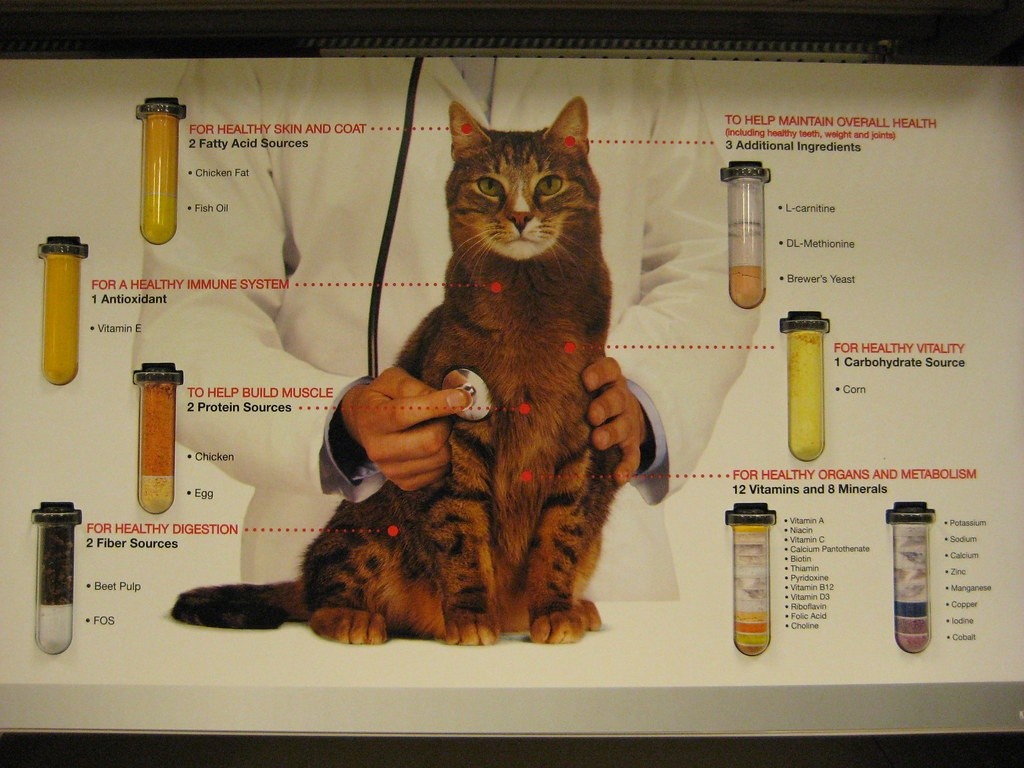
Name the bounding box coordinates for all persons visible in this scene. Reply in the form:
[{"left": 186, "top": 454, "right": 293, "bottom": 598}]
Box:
[{"left": 132, "top": 57, "right": 757, "bottom": 603}]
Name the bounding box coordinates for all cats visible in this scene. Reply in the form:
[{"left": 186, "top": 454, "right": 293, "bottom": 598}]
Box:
[{"left": 171, "top": 96, "right": 623, "bottom": 644}]
